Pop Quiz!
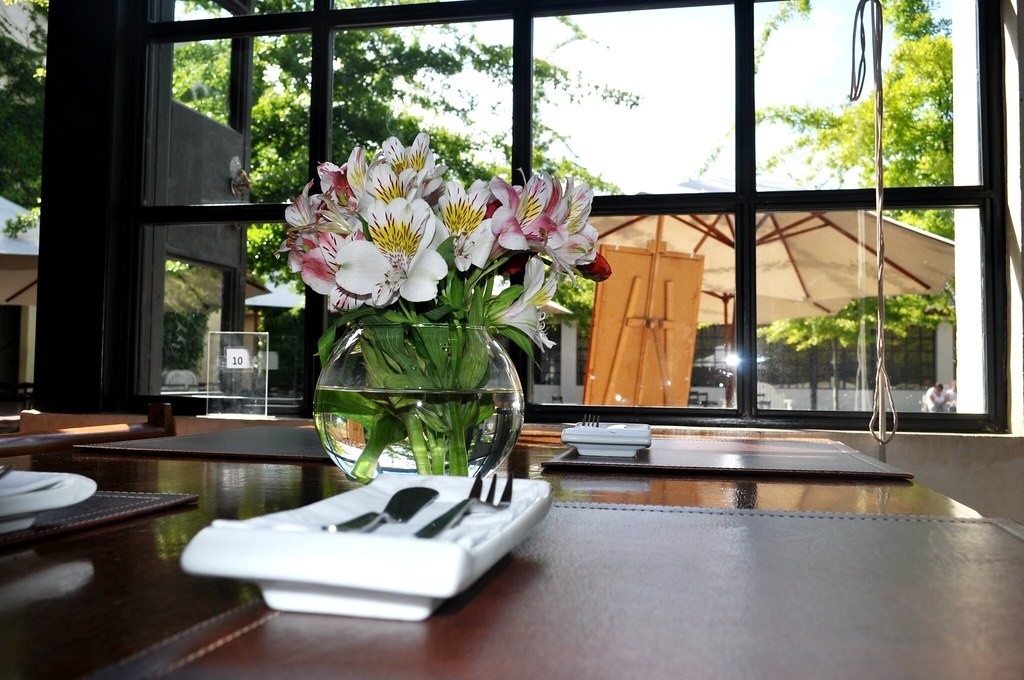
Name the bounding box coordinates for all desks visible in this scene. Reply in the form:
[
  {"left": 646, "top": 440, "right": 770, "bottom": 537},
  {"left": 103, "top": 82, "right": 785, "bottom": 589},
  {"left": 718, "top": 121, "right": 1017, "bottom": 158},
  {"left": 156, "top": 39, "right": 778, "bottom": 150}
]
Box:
[{"left": 0, "top": 425, "right": 1024, "bottom": 680}]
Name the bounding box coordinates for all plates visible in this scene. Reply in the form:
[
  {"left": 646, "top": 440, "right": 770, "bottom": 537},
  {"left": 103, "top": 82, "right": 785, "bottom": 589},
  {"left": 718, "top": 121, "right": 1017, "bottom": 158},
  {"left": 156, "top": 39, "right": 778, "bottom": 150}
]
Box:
[
  {"left": 0, "top": 471, "right": 96, "bottom": 516},
  {"left": 561, "top": 423, "right": 651, "bottom": 457},
  {"left": 179, "top": 473, "right": 554, "bottom": 624}
]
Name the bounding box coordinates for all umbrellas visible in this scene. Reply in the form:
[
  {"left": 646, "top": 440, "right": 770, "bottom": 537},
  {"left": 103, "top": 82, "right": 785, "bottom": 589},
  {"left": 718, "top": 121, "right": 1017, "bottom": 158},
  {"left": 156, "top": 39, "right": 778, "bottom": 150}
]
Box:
[
  {"left": 586, "top": 191, "right": 954, "bottom": 411},
  {"left": 0, "top": 261, "right": 273, "bottom": 315}
]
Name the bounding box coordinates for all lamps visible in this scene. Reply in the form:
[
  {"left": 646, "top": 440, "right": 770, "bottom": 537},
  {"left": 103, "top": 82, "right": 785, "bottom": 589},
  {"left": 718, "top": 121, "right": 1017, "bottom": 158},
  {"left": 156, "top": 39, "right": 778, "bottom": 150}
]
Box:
[{"left": 230, "top": 156, "right": 253, "bottom": 196}]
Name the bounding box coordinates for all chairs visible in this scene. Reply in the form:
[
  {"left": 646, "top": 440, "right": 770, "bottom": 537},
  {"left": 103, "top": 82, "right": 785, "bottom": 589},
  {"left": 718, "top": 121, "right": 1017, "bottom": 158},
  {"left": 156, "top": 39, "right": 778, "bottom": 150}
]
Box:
[
  {"left": 12, "top": 382, "right": 35, "bottom": 413},
  {"left": 165, "top": 370, "right": 199, "bottom": 391},
  {"left": 688, "top": 391, "right": 772, "bottom": 408}
]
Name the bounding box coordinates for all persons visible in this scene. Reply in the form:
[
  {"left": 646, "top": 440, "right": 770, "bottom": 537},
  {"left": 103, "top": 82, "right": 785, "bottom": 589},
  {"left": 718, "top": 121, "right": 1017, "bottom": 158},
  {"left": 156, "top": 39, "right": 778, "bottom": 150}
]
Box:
[
  {"left": 924, "top": 383, "right": 948, "bottom": 412},
  {"left": 946, "top": 381, "right": 957, "bottom": 412}
]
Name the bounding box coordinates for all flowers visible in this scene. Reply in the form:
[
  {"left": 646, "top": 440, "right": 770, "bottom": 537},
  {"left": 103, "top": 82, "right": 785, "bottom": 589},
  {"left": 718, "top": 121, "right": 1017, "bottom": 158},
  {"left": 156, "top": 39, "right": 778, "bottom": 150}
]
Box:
[{"left": 274, "top": 130, "right": 613, "bottom": 479}]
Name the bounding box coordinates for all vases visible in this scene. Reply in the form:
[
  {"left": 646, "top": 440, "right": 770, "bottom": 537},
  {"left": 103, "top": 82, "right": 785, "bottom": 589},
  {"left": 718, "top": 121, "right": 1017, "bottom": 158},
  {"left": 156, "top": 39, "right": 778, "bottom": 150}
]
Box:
[{"left": 312, "top": 323, "right": 526, "bottom": 485}]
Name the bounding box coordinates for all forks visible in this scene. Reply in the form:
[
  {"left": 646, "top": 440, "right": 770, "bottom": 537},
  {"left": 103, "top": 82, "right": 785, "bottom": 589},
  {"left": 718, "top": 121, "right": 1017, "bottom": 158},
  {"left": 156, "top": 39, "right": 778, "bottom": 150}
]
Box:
[
  {"left": 414, "top": 472, "right": 513, "bottom": 538},
  {"left": 582, "top": 414, "right": 600, "bottom": 427}
]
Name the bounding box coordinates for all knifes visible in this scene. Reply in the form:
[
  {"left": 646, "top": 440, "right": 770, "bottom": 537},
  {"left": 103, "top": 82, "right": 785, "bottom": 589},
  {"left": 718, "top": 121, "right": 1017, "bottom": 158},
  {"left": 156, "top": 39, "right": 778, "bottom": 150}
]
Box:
[{"left": 320, "top": 486, "right": 440, "bottom": 534}]
